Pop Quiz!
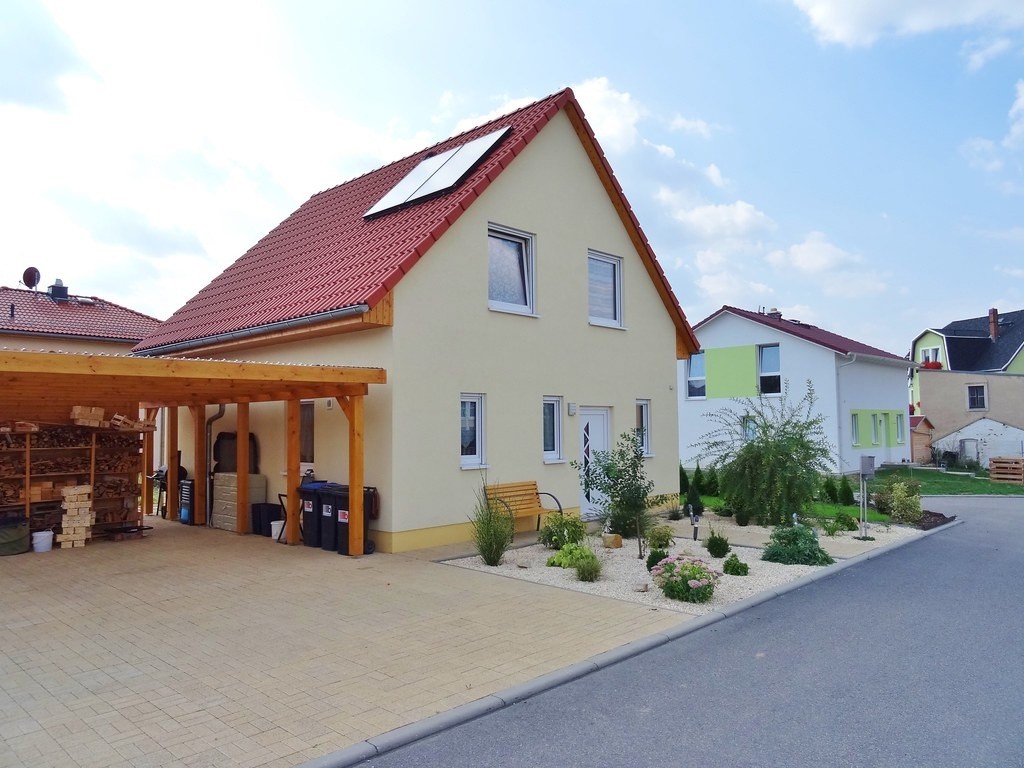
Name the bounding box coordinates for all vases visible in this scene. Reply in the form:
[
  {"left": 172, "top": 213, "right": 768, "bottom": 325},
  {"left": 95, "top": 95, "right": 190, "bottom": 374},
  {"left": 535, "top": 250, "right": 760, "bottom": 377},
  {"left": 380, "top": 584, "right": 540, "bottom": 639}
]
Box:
[{"left": 910, "top": 412, "right": 914, "bottom": 415}]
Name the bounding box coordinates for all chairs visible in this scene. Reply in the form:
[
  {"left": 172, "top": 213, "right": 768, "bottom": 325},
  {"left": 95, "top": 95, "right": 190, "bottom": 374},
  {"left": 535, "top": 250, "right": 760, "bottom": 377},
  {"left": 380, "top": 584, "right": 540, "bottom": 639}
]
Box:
[{"left": 276, "top": 476, "right": 315, "bottom": 545}]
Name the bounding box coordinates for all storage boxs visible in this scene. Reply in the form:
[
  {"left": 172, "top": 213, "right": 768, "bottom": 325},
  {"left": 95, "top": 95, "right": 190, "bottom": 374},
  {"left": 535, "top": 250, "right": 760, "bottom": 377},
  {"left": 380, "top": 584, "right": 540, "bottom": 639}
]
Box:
[
  {"left": 19, "top": 478, "right": 96, "bottom": 549},
  {"left": 70, "top": 405, "right": 105, "bottom": 426},
  {"left": 213, "top": 474, "right": 266, "bottom": 533},
  {"left": 270, "top": 520, "right": 303, "bottom": 539}
]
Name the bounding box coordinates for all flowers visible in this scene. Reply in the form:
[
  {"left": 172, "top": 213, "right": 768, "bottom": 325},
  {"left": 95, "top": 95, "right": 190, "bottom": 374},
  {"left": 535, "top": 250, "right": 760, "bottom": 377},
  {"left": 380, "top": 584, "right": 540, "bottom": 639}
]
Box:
[
  {"left": 923, "top": 361, "right": 942, "bottom": 369},
  {"left": 909, "top": 404, "right": 914, "bottom": 411}
]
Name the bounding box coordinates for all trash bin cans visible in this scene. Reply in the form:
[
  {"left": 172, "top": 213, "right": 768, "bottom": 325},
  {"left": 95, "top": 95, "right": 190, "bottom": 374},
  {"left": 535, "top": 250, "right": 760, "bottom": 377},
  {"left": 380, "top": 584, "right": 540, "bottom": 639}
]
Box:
[
  {"left": 297, "top": 481, "right": 328, "bottom": 547},
  {"left": 318, "top": 482, "right": 349, "bottom": 552},
  {"left": 336, "top": 485, "right": 378, "bottom": 556}
]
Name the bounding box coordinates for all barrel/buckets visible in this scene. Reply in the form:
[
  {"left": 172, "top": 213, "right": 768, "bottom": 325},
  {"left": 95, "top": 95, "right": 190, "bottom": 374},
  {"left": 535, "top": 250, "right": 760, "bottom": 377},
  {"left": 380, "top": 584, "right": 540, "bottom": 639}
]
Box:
[
  {"left": 32, "top": 529, "right": 54, "bottom": 552},
  {"left": 271, "top": 521, "right": 288, "bottom": 540}
]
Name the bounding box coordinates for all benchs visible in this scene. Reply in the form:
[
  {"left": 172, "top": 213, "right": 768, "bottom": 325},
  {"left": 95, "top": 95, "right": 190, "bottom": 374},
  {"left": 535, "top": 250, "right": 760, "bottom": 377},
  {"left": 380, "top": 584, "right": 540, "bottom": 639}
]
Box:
[{"left": 484, "top": 479, "right": 563, "bottom": 543}]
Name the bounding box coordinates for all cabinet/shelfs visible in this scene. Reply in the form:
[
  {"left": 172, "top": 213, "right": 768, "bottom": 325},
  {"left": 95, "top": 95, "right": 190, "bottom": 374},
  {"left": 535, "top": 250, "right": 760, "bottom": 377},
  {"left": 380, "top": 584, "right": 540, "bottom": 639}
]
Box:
[
  {"left": 0, "top": 427, "right": 157, "bottom": 539},
  {"left": 178, "top": 479, "right": 213, "bottom": 526}
]
